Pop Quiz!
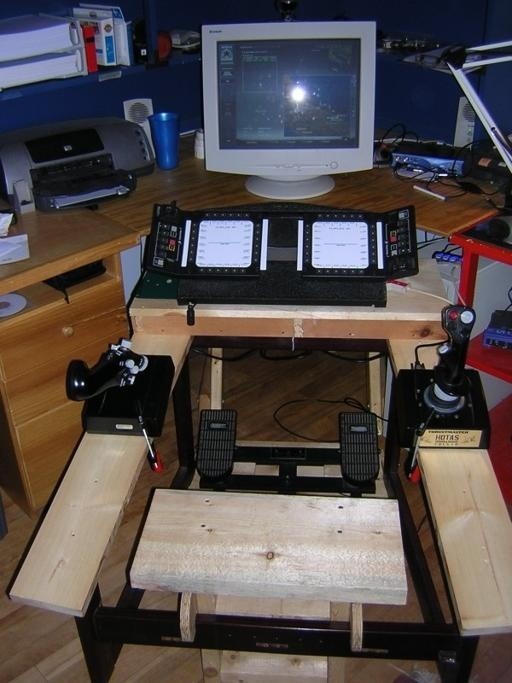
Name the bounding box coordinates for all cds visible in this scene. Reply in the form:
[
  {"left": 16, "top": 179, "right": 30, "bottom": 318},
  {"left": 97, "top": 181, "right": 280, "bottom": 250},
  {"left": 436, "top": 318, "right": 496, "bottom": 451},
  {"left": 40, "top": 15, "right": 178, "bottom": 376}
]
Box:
[{"left": 0, "top": 293, "right": 27, "bottom": 317}]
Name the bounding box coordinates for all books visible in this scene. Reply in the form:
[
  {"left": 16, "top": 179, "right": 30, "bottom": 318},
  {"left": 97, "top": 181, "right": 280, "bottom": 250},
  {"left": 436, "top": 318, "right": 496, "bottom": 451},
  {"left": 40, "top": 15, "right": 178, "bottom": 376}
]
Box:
[{"left": 0, "top": 12, "right": 83, "bottom": 87}]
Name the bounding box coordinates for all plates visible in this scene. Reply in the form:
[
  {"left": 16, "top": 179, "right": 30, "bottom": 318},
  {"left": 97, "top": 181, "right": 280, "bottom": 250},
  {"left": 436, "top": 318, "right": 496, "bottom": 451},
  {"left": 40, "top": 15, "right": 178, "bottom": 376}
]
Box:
[{"left": 171, "top": 41, "right": 200, "bottom": 49}]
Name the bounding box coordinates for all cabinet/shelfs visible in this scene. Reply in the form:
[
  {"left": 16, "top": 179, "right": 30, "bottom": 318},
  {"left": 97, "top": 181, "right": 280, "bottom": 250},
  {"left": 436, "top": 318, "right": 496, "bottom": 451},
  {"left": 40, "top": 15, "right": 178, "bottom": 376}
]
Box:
[{"left": 0, "top": 209, "right": 140, "bottom": 520}]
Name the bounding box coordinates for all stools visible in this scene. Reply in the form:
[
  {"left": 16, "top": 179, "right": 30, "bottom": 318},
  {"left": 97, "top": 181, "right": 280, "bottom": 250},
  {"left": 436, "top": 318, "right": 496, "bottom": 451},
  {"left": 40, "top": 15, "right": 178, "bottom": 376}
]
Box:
[{"left": 127, "top": 486, "right": 410, "bottom": 655}]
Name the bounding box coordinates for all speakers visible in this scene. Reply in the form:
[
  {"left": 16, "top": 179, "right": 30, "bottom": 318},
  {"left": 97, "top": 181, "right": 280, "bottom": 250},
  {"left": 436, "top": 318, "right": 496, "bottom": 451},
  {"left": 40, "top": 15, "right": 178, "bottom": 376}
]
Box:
[
  {"left": 123, "top": 98, "right": 157, "bottom": 160},
  {"left": 453, "top": 97, "right": 477, "bottom": 150}
]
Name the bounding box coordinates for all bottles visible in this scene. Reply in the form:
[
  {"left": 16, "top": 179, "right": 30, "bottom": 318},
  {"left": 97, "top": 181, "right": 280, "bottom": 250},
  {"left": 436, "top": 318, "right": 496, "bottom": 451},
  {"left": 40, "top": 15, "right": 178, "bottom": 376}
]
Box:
[{"left": 193, "top": 128, "right": 205, "bottom": 159}]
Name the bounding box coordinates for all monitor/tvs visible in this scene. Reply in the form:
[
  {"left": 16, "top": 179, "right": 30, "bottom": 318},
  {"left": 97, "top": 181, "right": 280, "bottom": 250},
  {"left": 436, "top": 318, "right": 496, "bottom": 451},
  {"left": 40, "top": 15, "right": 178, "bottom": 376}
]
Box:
[
  {"left": 302, "top": 212, "right": 376, "bottom": 278},
  {"left": 202, "top": 21, "right": 376, "bottom": 199},
  {"left": 188, "top": 212, "right": 261, "bottom": 277}
]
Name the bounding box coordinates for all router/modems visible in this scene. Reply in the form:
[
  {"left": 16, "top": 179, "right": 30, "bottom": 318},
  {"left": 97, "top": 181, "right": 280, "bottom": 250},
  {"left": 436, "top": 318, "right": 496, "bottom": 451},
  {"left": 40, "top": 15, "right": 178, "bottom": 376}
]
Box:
[{"left": 392, "top": 140, "right": 471, "bottom": 177}]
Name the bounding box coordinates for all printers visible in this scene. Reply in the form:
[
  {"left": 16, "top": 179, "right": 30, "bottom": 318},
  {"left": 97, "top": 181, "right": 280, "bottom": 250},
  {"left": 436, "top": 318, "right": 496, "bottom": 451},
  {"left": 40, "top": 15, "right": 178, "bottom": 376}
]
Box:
[{"left": 0, "top": 116, "right": 155, "bottom": 215}]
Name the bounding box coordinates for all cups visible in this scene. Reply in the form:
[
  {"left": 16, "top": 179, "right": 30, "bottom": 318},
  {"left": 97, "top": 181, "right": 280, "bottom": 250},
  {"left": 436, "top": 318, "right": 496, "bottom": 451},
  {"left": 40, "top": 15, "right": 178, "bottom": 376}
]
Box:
[{"left": 148, "top": 110, "right": 183, "bottom": 171}]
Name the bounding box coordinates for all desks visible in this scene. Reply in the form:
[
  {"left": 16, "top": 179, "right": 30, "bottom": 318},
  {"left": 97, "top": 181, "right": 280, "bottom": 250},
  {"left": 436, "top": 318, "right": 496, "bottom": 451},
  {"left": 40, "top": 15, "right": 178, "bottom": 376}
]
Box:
[{"left": 92, "top": 144, "right": 512, "bottom": 435}]
